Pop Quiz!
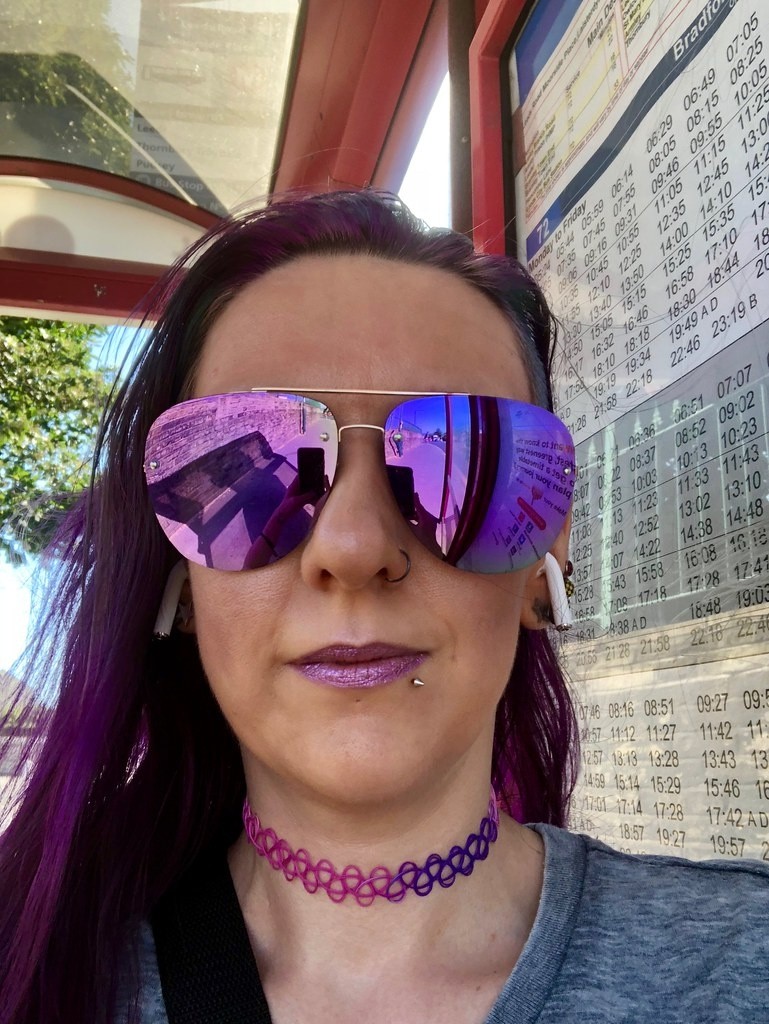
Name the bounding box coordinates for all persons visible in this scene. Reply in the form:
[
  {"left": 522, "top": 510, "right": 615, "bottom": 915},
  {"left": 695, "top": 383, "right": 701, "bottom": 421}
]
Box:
[
  {"left": 406, "top": 491, "right": 442, "bottom": 560},
  {"left": 240, "top": 474, "right": 331, "bottom": 572},
  {"left": 0, "top": 186, "right": 769, "bottom": 1024}
]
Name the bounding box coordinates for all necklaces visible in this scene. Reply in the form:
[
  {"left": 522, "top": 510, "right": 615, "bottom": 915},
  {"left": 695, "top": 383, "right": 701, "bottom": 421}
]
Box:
[{"left": 242, "top": 784, "right": 501, "bottom": 908}]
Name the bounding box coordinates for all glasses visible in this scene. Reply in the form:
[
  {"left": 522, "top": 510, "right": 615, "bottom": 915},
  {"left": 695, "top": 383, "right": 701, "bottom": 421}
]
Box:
[{"left": 138, "top": 386, "right": 576, "bottom": 577}]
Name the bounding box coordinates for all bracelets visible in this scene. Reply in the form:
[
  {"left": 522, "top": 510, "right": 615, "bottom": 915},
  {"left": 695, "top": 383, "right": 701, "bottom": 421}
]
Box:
[{"left": 260, "top": 533, "right": 277, "bottom": 557}]
